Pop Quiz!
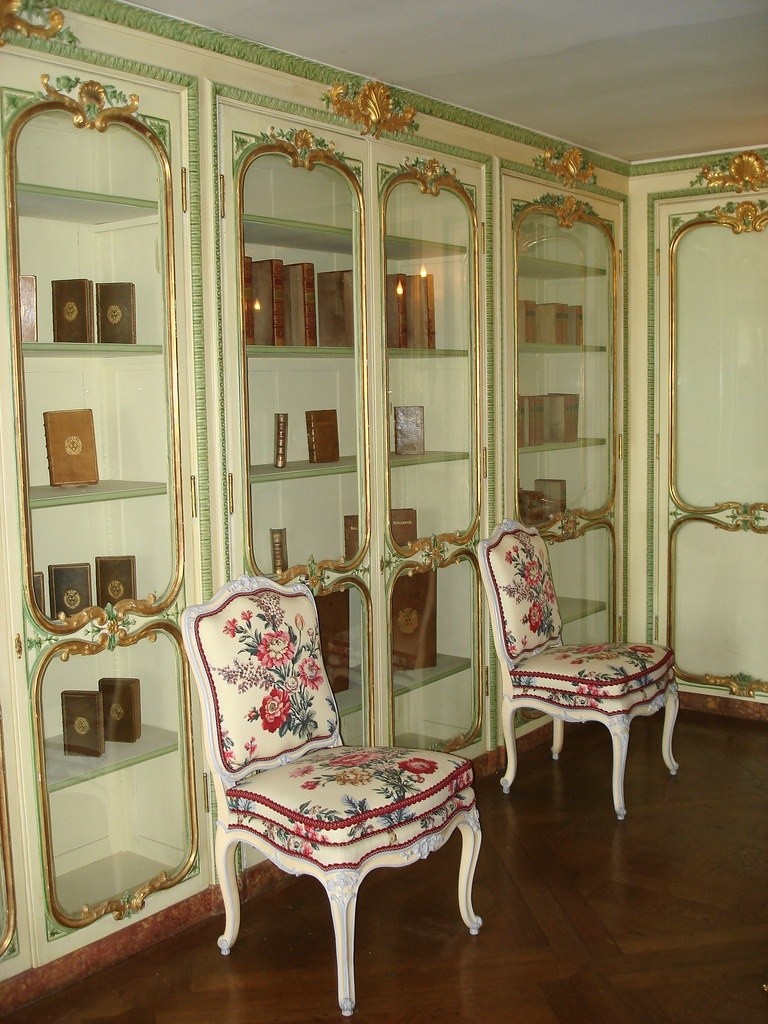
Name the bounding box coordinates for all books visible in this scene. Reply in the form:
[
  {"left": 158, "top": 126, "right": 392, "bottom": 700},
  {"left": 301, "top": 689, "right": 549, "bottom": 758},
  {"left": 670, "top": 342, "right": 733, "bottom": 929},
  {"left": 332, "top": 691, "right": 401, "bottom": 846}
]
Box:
[
  {"left": 344, "top": 514, "right": 359, "bottom": 560},
  {"left": 21, "top": 275, "right": 136, "bottom": 344},
  {"left": 315, "top": 587, "right": 350, "bottom": 696},
  {"left": 394, "top": 405, "right": 426, "bottom": 457},
  {"left": 43, "top": 409, "right": 99, "bottom": 488},
  {"left": 270, "top": 528, "right": 289, "bottom": 573},
  {"left": 274, "top": 412, "right": 288, "bottom": 469},
  {"left": 391, "top": 565, "right": 437, "bottom": 673},
  {"left": 517, "top": 393, "right": 579, "bottom": 448},
  {"left": 518, "top": 478, "right": 566, "bottom": 525},
  {"left": 304, "top": 409, "right": 339, "bottom": 464},
  {"left": 517, "top": 299, "right": 584, "bottom": 346},
  {"left": 243, "top": 256, "right": 436, "bottom": 349},
  {"left": 61, "top": 678, "right": 141, "bottom": 758},
  {"left": 390, "top": 508, "right": 417, "bottom": 548},
  {"left": 33, "top": 555, "right": 137, "bottom": 621}
]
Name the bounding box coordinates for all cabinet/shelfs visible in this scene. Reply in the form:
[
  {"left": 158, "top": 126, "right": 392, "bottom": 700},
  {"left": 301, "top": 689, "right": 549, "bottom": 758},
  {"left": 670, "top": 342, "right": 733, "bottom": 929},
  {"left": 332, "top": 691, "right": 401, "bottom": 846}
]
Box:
[
  {"left": 230, "top": 208, "right": 480, "bottom": 739},
  {"left": 16, "top": 177, "right": 179, "bottom": 796},
  {"left": 513, "top": 248, "right": 613, "bottom": 643}
]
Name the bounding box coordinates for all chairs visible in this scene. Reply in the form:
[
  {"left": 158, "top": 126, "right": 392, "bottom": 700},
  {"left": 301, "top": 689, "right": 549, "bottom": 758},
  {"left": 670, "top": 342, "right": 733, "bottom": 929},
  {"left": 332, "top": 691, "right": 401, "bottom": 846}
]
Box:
[
  {"left": 182, "top": 575, "right": 481, "bottom": 1017},
  {"left": 474, "top": 519, "right": 681, "bottom": 821}
]
